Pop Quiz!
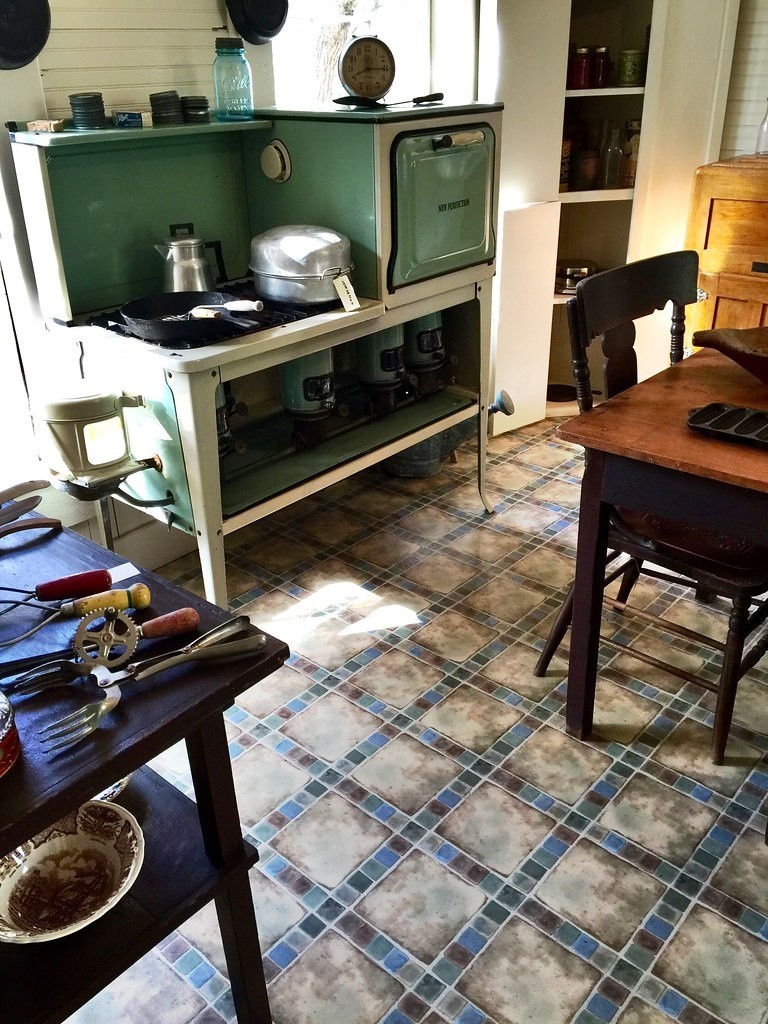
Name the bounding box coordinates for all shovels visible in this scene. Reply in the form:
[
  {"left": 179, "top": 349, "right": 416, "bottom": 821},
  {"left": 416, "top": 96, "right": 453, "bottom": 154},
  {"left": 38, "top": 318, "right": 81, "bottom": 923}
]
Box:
[
  {"left": 159, "top": 299, "right": 264, "bottom": 322},
  {"left": 333, "top": 93, "right": 444, "bottom": 108}
]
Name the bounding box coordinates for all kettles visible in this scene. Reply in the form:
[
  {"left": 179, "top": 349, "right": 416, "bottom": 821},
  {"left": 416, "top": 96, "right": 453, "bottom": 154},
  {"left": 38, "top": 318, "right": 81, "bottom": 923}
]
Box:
[{"left": 154, "top": 222, "right": 229, "bottom": 293}]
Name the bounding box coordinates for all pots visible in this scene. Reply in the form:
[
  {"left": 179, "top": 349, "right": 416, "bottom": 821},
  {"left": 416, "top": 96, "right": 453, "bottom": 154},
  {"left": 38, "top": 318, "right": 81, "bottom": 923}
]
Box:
[
  {"left": 249, "top": 224, "right": 356, "bottom": 303},
  {"left": 0, "top": 0, "right": 51, "bottom": 71},
  {"left": 120, "top": 293, "right": 259, "bottom": 342},
  {"left": 225, "top": 0, "right": 289, "bottom": 45}
]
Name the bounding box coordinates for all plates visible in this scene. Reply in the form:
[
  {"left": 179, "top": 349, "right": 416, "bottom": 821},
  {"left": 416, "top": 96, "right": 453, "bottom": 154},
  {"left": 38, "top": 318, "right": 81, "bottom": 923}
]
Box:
[
  {"left": 69, "top": 92, "right": 107, "bottom": 130},
  {"left": 149, "top": 90, "right": 211, "bottom": 125}
]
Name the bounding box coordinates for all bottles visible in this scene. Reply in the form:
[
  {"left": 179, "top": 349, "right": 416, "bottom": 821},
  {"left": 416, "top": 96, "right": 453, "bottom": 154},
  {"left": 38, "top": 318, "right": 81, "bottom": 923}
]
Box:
[
  {"left": 210, "top": 37, "right": 255, "bottom": 121},
  {"left": 597, "top": 127, "right": 624, "bottom": 190}
]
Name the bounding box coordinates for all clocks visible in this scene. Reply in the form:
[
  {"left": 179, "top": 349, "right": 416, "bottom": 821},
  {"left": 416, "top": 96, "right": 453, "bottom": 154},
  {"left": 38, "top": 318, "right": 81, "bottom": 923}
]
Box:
[{"left": 336, "top": 33, "right": 396, "bottom": 112}]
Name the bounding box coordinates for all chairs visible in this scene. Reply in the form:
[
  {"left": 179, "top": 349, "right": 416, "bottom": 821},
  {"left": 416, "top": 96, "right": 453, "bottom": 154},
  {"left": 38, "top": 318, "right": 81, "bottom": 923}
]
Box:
[{"left": 532, "top": 250, "right": 768, "bottom": 766}]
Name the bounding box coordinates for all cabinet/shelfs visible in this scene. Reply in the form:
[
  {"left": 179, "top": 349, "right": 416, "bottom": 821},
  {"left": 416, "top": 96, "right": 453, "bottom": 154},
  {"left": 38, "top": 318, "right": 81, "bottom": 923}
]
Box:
[
  {"left": 683, "top": 153, "right": 768, "bottom": 359},
  {"left": 430, "top": 0, "right": 742, "bottom": 438},
  {"left": 51, "top": 261, "right": 494, "bottom": 612},
  {"left": 0, "top": 496, "right": 291, "bottom": 1024}
]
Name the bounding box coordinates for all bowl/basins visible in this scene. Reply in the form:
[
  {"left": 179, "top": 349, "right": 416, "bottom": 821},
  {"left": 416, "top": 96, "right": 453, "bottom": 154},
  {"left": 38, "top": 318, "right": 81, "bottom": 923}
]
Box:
[{"left": 0, "top": 799, "right": 151, "bottom": 946}]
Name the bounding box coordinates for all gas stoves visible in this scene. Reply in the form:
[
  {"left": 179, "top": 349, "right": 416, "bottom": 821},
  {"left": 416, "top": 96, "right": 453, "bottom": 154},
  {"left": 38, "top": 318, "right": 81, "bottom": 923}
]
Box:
[{"left": 86, "top": 278, "right": 354, "bottom": 348}]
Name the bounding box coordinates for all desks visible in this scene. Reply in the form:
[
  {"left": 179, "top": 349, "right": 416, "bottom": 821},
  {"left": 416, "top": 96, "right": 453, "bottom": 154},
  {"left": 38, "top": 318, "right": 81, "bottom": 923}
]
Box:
[{"left": 556, "top": 346, "right": 768, "bottom": 740}]
